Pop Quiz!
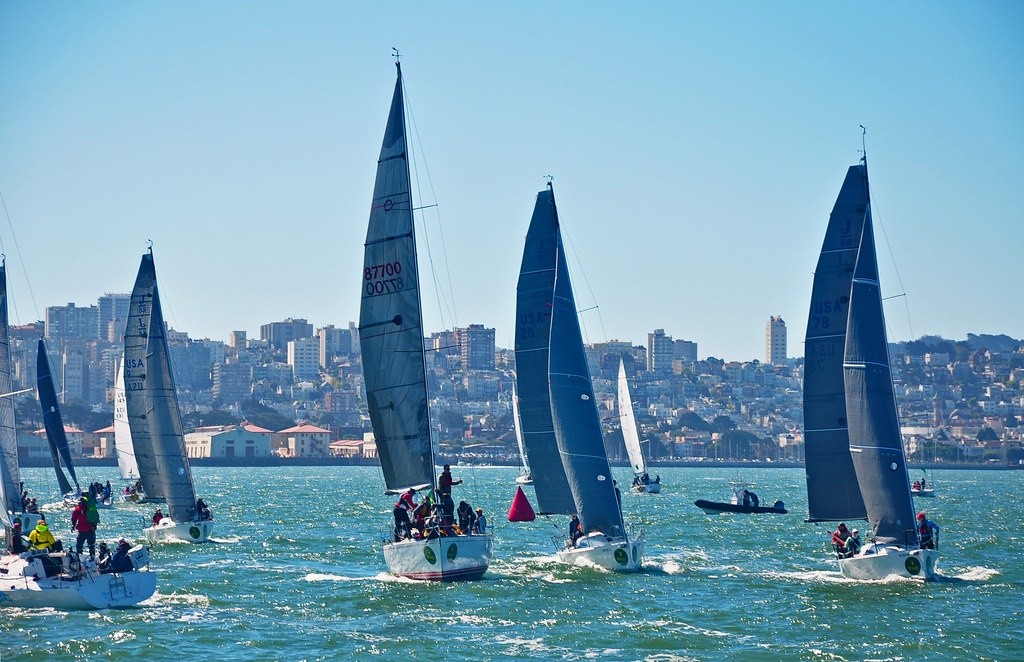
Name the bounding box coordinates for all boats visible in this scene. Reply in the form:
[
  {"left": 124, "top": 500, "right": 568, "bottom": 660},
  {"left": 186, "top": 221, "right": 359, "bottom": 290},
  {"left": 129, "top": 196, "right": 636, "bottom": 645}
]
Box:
[
  {"left": 911, "top": 484, "right": 935, "bottom": 497},
  {"left": 693, "top": 500, "right": 788, "bottom": 514},
  {"left": 0, "top": 542, "right": 158, "bottom": 611},
  {"left": 728, "top": 477, "right": 756, "bottom": 487}
]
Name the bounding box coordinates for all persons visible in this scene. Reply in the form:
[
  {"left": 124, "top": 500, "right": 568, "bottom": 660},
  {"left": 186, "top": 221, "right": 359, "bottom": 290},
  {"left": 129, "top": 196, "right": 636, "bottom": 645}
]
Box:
[
  {"left": 197, "top": 498, "right": 210, "bottom": 520},
  {"left": 831, "top": 522, "right": 852, "bottom": 559},
  {"left": 432, "top": 464, "right": 486, "bottom": 537},
  {"left": 632, "top": 473, "right": 660, "bottom": 488},
  {"left": 569, "top": 514, "right": 584, "bottom": 541},
  {"left": 99, "top": 543, "right": 111, "bottom": 569},
  {"left": 10, "top": 523, "right": 27, "bottom": 554},
  {"left": 153, "top": 508, "right": 163, "bottom": 525},
  {"left": 743, "top": 490, "right": 759, "bottom": 507},
  {"left": 913, "top": 477, "right": 926, "bottom": 491},
  {"left": 413, "top": 495, "right": 432, "bottom": 519},
  {"left": 70, "top": 498, "right": 97, "bottom": 561},
  {"left": 393, "top": 488, "right": 418, "bottom": 543},
  {"left": 20, "top": 491, "right": 39, "bottom": 514},
  {"left": 28, "top": 520, "right": 63, "bottom": 551},
  {"left": 916, "top": 512, "right": 941, "bottom": 550},
  {"left": 109, "top": 538, "right": 134, "bottom": 572},
  {"left": 844, "top": 529, "right": 863, "bottom": 557},
  {"left": 88, "top": 480, "right": 111, "bottom": 503},
  {"left": 124, "top": 479, "right": 142, "bottom": 494}
]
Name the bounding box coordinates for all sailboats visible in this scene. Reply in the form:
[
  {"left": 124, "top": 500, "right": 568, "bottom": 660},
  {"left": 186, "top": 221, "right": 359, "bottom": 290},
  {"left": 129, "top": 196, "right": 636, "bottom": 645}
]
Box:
[
  {"left": 617, "top": 352, "right": 663, "bottom": 494},
  {"left": 357, "top": 43, "right": 496, "bottom": 583},
  {"left": 123, "top": 239, "right": 215, "bottom": 546},
  {"left": 0, "top": 254, "right": 44, "bottom": 539},
  {"left": 511, "top": 379, "right": 534, "bottom": 484},
  {"left": 802, "top": 124, "right": 940, "bottom": 581},
  {"left": 37, "top": 337, "right": 115, "bottom": 514},
  {"left": 110, "top": 351, "right": 168, "bottom": 504},
  {"left": 506, "top": 173, "right": 649, "bottom": 574}
]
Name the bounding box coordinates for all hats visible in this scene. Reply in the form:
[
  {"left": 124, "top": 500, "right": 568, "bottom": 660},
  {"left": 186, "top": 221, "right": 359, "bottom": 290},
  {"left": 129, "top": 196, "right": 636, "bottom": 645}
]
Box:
[
  {"left": 852, "top": 529, "right": 858, "bottom": 533},
  {"left": 78, "top": 497, "right": 88, "bottom": 503},
  {"left": 14, "top": 523, "right": 20, "bottom": 528},
  {"left": 476, "top": 508, "right": 484, "bottom": 514},
  {"left": 917, "top": 513, "right": 925, "bottom": 520},
  {"left": 37, "top": 520, "right": 44, "bottom": 525},
  {"left": 408, "top": 488, "right": 417, "bottom": 495}
]
